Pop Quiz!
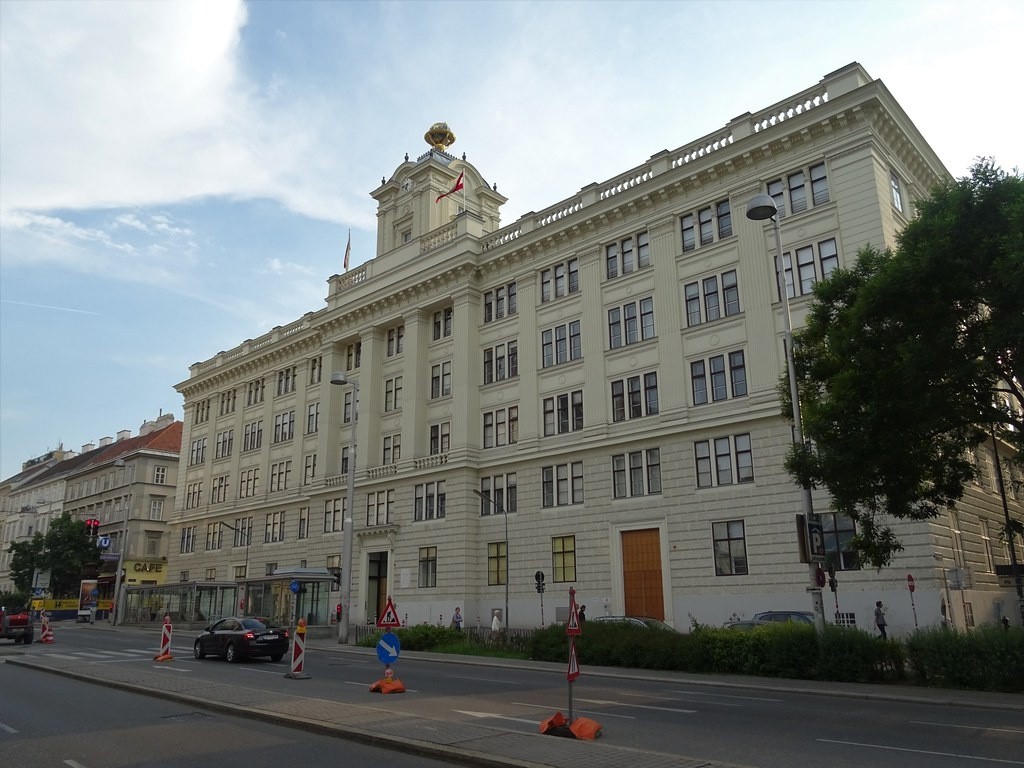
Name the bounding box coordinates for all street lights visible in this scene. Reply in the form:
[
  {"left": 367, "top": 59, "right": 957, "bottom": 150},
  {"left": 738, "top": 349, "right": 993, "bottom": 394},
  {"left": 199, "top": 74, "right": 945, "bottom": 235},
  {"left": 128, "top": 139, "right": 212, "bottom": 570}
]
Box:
[
  {"left": 473, "top": 489, "right": 509, "bottom": 629},
  {"left": 219, "top": 522, "right": 248, "bottom": 579},
  {"left": 330, "top": 380, "right": 357, "bottom": 644},
  {"left": 111, "top": 458, "right": 132, "bottom": 626},
  {"left": 35, "top": 572, "right": 43, "bottom": 587},
  {"left": 36, "top": 497, "right": 53, "bottom": 554},
  {"left": 745, "top": 195, "right": 830, "bottom": 679}
]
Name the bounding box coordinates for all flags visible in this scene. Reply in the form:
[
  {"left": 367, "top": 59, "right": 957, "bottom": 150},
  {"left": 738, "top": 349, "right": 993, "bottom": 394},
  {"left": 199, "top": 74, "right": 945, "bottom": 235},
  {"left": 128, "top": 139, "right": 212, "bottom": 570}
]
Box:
[
  {"left": 344, "top": 239, "right": 350, "bottom": 268},
  {"left": 436, "top": 172, "right": 463, "bottom": 204}
]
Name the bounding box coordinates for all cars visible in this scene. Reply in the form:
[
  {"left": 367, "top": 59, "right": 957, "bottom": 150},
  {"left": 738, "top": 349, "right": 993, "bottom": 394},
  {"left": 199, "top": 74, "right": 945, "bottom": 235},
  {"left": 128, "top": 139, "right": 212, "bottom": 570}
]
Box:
[
  {"left": 718, "top": 620, "right": 779, "bottom": 632},
  {"left": 593, "top": 616, "right": 675, "bottom": 631},
  {"left": 194, "top": 617, "right": 289, "bottom": 663}
]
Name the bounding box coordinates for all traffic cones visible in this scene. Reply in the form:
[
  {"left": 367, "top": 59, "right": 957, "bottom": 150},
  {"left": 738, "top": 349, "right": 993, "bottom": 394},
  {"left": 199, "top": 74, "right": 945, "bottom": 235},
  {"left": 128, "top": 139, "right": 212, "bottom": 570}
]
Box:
[{"left": 45, "top": 627, "right": 54, "bottom": 643}]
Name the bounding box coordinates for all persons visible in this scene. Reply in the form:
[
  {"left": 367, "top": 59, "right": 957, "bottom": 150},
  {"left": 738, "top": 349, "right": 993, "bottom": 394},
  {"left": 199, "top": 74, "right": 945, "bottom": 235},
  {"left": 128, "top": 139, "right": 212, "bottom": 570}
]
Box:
[
  {"left": 491, "top": 610, "right": 500, "bottom": 631},
  {"left": 453, "top": 607, "right": 463, "bottom": 631},
  {"left": 579, "top": 605, "right": 585, "bottom": 621},
  {"left": 874, "top": 601, "right": 887, "bottom": 639}
]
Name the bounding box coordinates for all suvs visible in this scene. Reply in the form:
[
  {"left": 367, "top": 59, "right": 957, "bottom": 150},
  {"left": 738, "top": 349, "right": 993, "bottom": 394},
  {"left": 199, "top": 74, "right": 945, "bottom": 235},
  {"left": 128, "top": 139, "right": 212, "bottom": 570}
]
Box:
[{"left": 754, "top": 610, "right": 833, "bottom": 626}]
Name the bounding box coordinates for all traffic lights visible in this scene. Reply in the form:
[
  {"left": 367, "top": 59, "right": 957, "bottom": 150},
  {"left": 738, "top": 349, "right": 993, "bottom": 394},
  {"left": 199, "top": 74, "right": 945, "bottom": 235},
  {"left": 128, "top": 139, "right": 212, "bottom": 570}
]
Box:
[
  {"left": 336, "top": 604, "right": 341, "bottom": 621},
  {"left": 84, "top": 519, "right": 92, "bottom": 536},
  {"left": 109, "top": 603, "right": 113, "bottom": 613},
  {"left": 92, "top": 520, "right": 100, "bottom": 536}
]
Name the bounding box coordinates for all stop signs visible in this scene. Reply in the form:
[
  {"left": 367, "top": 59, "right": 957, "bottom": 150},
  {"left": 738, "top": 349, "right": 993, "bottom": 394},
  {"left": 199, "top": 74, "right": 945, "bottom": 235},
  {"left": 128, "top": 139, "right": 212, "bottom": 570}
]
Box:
[{"left": 907, "top": 575, "right": 915, "bottom": 592}]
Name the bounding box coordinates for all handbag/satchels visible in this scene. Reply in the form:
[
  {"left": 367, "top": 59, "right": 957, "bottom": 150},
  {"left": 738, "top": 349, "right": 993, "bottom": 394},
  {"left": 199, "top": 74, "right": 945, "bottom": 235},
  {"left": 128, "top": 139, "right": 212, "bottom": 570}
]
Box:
[
  {"left": 450, "top": 615, "right": 457, "bottom": 630},
  {"left": 883, "top": 617, "right": 887, "bottom": 626}
]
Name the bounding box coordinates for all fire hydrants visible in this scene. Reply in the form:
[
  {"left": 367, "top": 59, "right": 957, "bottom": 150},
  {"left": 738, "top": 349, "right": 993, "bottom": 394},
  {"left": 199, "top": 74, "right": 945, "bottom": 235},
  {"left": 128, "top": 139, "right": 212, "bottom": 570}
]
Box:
[{"left": 89, "top": 602, "right": 97, "bottom": 624}]
